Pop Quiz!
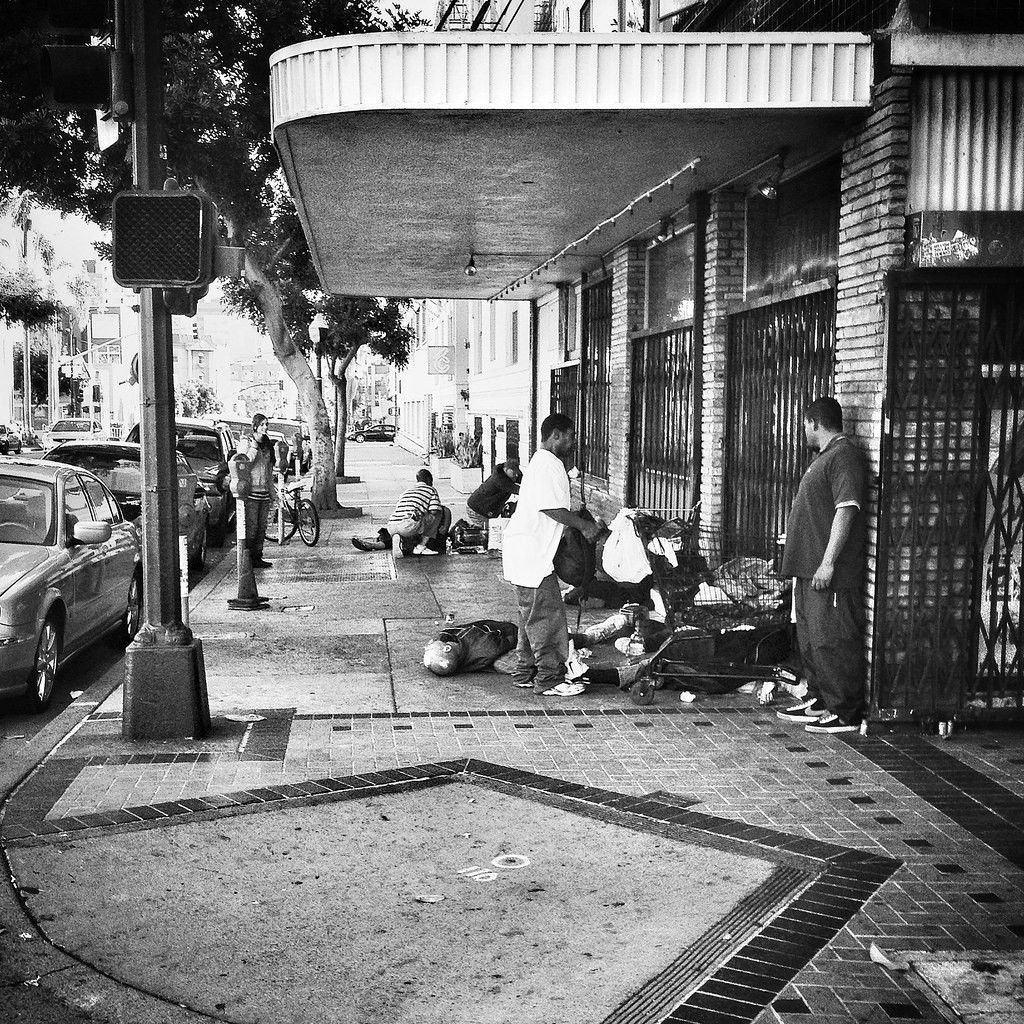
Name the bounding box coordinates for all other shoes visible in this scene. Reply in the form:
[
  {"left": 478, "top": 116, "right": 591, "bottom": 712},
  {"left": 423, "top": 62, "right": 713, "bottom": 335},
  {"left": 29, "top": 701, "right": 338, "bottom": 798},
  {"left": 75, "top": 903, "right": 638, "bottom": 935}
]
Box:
[
  {"left": 253, "top": 559, "right": 271, "bottom": 568},
  {"left": 352, "top": 538, "right": 372, "bottom": 551},
  {"left": 392, "top": 534, "right": 404, "bottom": 559},
  {"left": 542, "top": 680, "right": 585, "bottom": 696},
  {"left": 413, "top": 544, "right": 439, "bottom": 554},
  {"left": 586, "top": 614, "right": 627, "bottom": 643},
  {"left": 516, "top": 670, "right": 537, "bottom": 687},
  {"left": 259, "top": 559, "right": 274, "bottom": 566}
]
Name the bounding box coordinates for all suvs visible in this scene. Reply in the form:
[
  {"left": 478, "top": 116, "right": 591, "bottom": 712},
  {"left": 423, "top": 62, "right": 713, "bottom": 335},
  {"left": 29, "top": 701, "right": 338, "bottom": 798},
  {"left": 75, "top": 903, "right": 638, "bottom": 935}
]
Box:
[{"left": 125, "top": 418, "right": 236, "bottom": 548}]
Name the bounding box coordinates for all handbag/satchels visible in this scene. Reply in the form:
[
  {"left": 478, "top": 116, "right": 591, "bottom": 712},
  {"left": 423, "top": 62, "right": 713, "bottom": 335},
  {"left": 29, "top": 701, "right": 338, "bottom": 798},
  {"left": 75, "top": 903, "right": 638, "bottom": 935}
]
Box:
[{"left": 553, "top": 506, "right": 597, "bottom": 587}]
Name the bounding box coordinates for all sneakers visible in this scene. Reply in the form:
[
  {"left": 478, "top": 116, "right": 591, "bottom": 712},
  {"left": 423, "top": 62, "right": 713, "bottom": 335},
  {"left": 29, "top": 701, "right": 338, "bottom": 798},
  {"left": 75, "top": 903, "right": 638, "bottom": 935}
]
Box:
[
  {"left": 805, "top": 711, "right": 860, "bottom": 733},
  {"left": 777, "top": 697, "right": 826, "bottom": 722}
]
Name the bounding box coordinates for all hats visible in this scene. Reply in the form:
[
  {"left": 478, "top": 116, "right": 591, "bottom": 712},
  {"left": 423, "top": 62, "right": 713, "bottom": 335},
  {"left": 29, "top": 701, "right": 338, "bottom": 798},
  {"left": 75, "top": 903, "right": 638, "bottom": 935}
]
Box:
[{"left": 504, "top": 458, "right": 521, "bottom": 474}]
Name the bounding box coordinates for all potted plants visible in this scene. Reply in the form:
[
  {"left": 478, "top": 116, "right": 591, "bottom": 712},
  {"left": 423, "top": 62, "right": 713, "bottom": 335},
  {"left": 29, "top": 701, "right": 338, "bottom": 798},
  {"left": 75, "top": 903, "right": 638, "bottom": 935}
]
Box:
[
  {"left": 430, "top": 428, "right": 454, "bottom": 479},
  {"left": 449, "top": 433, "right": 483, "bottom": 494}
]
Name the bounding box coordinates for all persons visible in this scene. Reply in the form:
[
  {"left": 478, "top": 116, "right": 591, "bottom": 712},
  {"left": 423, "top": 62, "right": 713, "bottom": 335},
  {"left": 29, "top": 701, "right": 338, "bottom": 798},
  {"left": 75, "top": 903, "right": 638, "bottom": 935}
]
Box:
[
  {"left": 498, "top": 414, "right": 602, "bottom": 698},
  {"left": 448, "top": 459, "right": 524, "bottom": 539},
  {"left": 351, "top": 504, "right": 451, "bottom": 552},
  {"left": 237, "top": 413, "right": 275, "bottom": 568},
  {"left": 776, "top": 395, "right": 872, "bottom": 736},
  {"left": 421, "top": 615, "right": 630, "bottom": 676},
  {"left": 385, "top": 469, "right": 445, "bottom": 560},
  {"left": 563, "top": 625, "right": 795, "bottom": 706}
]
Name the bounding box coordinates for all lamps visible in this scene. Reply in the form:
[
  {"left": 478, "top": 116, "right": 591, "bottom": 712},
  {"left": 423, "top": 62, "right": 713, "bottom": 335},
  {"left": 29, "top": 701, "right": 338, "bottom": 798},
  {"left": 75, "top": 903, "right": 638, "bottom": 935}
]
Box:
[
  {"left": 463, "top": 255, "right": 477, "bottom": 276},
  {"left": 756, "top": 164, "right": 785, "bottom": 200}
]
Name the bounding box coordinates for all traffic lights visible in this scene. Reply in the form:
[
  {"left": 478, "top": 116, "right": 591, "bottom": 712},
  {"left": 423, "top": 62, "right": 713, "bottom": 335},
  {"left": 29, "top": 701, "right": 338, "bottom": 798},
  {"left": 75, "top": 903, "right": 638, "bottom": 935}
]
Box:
[
  {"left": 95, "top": 406, "right": 100, "bottom": 412},
  {"left": 112, "top": 189, "right": 214, "bottom": 289},
  {"left": 78, "top": 389, "right": 84, "bottom": 402},
  {"left": 192, "top": 323, "right": 198, "bottom": 339},
  {"left": 83, "top": 407, "right": 89, "bottom": 412}
]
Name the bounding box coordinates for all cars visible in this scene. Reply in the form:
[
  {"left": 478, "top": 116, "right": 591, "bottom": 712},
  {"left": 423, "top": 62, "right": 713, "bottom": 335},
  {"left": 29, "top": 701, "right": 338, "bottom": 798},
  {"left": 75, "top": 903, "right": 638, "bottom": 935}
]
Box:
[
  {"left": 347, "top": 425, "right": 400, "bottom": 443},
  {"left": 200, "top": 415, "right": 312, "bottom": 483},
  {"left": 39, "top": 441, "right": 211, "bottom": 574},
  {"left": 44, "top": 418, "right": 107, "bottom": 455},
  {"left": 0, "top": 456, "right": 142, "bottom": 715},
  {"left": 0, "top": 424, "right": 23, "bottom": 455}
]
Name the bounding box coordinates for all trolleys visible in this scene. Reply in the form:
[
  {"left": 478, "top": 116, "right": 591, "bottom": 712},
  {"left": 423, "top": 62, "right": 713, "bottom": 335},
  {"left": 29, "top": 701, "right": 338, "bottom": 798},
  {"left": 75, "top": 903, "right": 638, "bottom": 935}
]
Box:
[{"left": 625, "top": 510, "right": 801, "bottom": 706}]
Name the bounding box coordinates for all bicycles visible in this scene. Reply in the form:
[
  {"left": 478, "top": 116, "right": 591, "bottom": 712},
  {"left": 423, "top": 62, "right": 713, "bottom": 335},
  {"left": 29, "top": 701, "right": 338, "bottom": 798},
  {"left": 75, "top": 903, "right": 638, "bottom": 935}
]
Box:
[{"left": 265, "top": 469, "right": 321, "bottom": 547}]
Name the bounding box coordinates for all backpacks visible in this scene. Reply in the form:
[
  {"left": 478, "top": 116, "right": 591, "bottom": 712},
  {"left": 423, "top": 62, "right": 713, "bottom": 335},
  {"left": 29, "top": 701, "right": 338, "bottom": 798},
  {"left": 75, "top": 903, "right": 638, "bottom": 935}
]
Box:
[{"left": 216, "top": 462, "right": 231, "bottom": 493}]
